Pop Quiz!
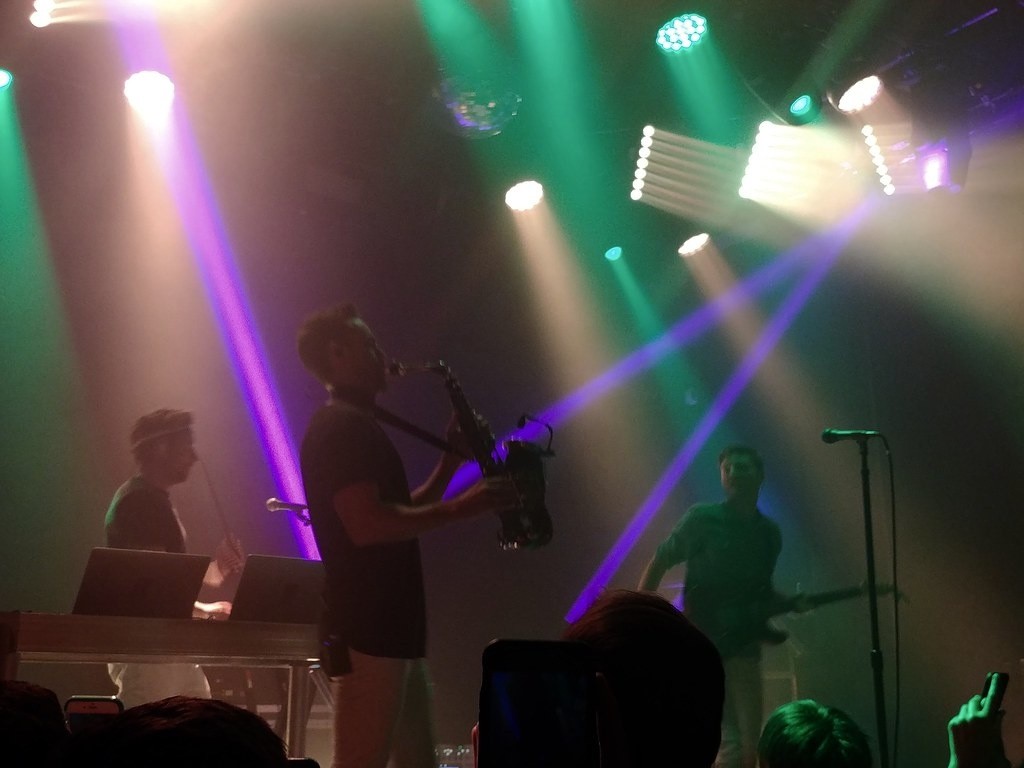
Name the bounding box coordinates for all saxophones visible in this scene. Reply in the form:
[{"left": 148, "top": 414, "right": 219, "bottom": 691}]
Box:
[{"left": 383, "top": 358, "right": 554, "bottom": 553}]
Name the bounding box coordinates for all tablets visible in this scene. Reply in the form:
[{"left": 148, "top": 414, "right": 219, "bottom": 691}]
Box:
[{"left": 475, "top": 635, "right": 607, "bottom": 767}]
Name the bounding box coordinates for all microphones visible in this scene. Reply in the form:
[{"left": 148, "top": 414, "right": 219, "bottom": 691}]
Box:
[
  {"left": 264, "top": 497, "right": 309, "bottom": 514},
  {"left": 820, "top": 425, "right": 880, "bottom": 445}
]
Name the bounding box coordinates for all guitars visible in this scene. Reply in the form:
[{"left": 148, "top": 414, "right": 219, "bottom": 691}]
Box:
[{"left": 680, "top": 577, "right": 912, "bottom": 662}]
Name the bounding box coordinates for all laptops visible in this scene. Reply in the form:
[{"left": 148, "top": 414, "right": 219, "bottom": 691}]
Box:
[
  {"left": 72, "top": 544, "right": 213, "bottom": 617},
  {"left": 227, "top": 553, "right": 329, "bottom": 624}
]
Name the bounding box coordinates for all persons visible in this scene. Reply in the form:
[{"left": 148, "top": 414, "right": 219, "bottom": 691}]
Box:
[
  {"left": 104, "top": 410, "right": 236, "bottom": 707},
  {"left": 296, "top": 308, "right": 525, "bottom": 768},
  {"left": 0, "top": 589, "right": 1024, "bottom": 768},
  {"left": 638, "top": 444, "right": 820, "bottom": 768}
]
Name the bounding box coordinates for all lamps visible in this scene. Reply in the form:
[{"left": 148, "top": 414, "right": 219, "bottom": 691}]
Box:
[
  {"left": 823, "top": 57, "right": 884, "bottom": 114},
  {"left": 736, "top": 46, "right": 822, "bottom": 127},
  {"left": 915, "top": 100, "right": 974, "bottom": 200}
]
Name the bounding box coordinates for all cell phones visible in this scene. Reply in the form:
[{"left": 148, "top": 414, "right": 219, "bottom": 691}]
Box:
[
  {"left": 64, "top": 696, "right": 125, "bottom": 736},
  {"left": 979, "top": 670, "right": 1010, "bottom": 710}
]
